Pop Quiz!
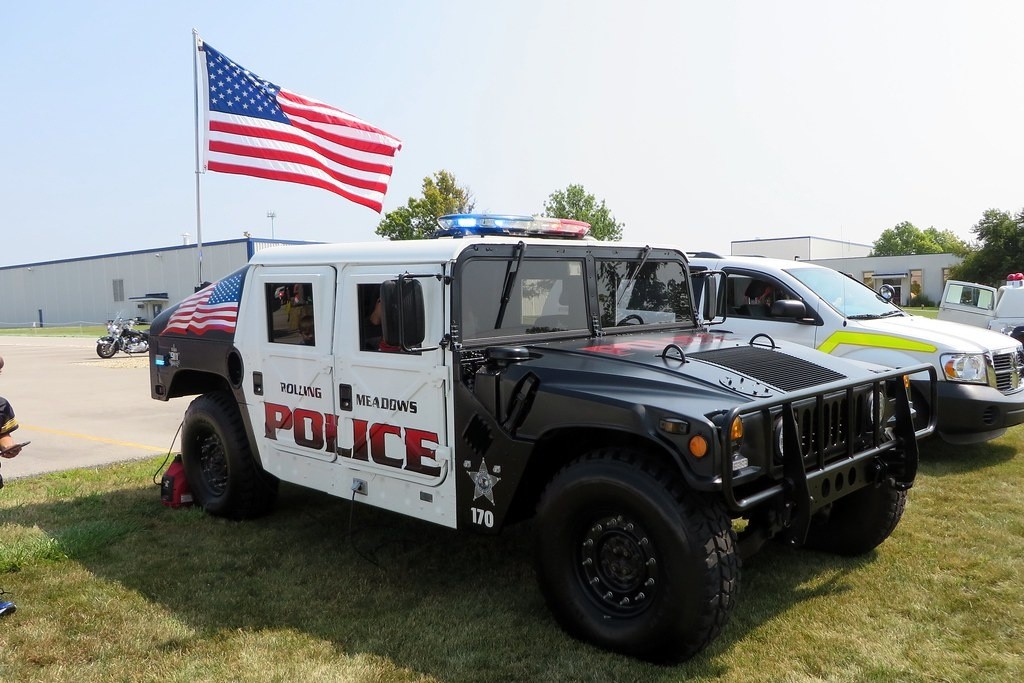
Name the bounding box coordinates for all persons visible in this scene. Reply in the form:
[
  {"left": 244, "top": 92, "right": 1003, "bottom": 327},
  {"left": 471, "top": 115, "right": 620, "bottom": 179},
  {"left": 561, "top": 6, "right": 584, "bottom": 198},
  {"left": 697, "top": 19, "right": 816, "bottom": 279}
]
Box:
[
  {"left": 296, "top": 315, "right": 316, "bottom": 347},
  {"left": 364, "top": 296, "right": 384, "bottom": 351},
  {"left": 0, "top": 357, "right": 23, "bottom": 619}
]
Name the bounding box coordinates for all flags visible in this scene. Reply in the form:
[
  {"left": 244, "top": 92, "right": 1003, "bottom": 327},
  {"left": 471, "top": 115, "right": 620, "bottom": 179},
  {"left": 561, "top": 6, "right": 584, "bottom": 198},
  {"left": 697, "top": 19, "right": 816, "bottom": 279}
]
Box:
[{"left": 196, "top": 33, "right": 403, "bottom": 212}]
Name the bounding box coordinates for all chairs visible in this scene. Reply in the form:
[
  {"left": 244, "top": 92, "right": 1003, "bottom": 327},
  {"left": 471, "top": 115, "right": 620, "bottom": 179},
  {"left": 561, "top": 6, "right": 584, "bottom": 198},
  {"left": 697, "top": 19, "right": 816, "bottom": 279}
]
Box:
[{"left": 739, "top": 279, "right": 770, "bottom": 318}]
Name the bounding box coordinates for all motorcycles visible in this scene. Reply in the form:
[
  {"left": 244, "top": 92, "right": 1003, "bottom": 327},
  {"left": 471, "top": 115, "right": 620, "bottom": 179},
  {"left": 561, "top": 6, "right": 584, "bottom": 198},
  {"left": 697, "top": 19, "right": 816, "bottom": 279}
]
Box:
[{"left": 95, "top": 310, "right": 150, "bottom": 359}]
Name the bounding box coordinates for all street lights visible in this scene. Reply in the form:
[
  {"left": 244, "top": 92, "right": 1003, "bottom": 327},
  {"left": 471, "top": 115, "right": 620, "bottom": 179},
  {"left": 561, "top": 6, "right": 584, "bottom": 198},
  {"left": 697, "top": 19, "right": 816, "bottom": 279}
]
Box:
[{"left": 267, "top": 211, "right": 277, "bottom": 239}]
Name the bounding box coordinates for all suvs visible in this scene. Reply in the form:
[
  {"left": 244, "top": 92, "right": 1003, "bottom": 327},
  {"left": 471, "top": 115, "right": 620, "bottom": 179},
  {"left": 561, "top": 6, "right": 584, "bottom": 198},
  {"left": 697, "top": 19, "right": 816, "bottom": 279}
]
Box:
[
  {"left": 540, "top": 251, "right": 1024, "bottom": 446},
  {"left": 150, "top": 212, "right": 921, "bottom": 666}
]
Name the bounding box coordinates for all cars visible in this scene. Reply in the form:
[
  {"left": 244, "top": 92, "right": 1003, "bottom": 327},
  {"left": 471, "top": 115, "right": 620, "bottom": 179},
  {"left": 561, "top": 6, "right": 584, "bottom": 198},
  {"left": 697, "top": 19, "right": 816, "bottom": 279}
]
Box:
[{"left": 938, "top": 272, "right": 1024, "bottom": 374}]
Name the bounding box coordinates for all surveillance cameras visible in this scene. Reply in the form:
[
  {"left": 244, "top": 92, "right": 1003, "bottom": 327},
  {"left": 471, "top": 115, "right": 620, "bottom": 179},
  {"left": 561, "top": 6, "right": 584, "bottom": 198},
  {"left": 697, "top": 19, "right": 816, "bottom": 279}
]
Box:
[{"left": 244, "top": 233, "right": 251, "bottom": 236}]
